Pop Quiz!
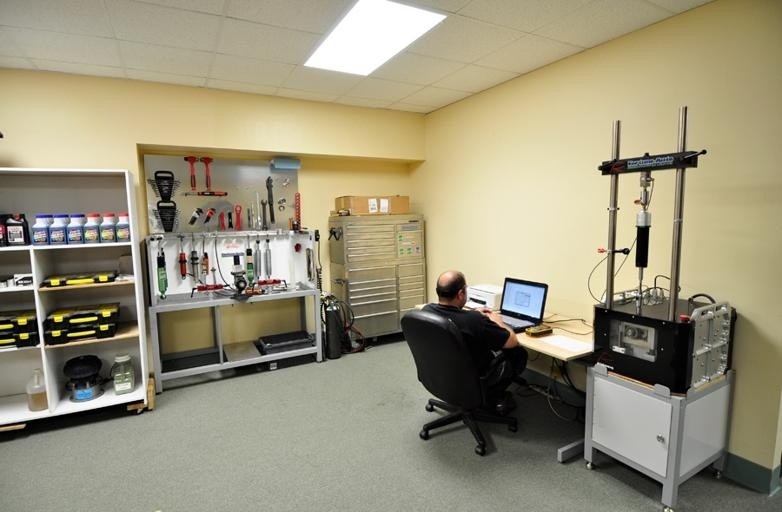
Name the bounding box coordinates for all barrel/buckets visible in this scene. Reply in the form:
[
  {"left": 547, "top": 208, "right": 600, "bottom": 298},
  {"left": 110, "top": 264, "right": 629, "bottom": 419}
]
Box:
[{"left": 31, "top": 212, "right": 130, "bottom": 245}]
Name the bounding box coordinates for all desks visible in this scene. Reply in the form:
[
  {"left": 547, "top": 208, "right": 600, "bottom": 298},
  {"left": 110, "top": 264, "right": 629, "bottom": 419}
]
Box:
[{"left": 415, "top": 302, "right": 595, "bottom": 462}]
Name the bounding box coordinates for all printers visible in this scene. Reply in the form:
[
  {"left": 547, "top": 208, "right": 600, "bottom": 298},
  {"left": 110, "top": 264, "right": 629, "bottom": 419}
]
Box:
[{"left": 464, "top": 284, "right": 503, "bottom": 311}]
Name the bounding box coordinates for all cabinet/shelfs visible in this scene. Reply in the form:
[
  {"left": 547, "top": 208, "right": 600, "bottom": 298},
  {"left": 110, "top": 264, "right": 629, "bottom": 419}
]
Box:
[
  {"left": 584, "top": 365, "right": 735, "bottom": 512},
  {"left": 329, "top": 213, "right": 427, "bottom": 343},
  {"left": 0, "top": 168, "right": 149, "bottom": 432},
  {"left": 144, "top": 229, "right": 322, "bottom": 396}
]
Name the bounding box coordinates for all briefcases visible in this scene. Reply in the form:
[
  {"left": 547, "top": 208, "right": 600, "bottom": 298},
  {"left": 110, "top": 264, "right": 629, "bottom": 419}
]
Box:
[{"left": 255, "top": 330, "right": 315, "bottom": 355}]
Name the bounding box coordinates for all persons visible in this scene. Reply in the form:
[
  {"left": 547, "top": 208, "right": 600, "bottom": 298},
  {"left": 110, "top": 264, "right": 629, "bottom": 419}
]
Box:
[{"left": 423, "top": 270, "right": 530, "bottom": 390}]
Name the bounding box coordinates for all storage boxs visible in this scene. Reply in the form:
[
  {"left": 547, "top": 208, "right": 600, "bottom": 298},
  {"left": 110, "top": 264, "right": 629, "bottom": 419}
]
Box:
[{"left": 335, "top": 196, "right": 409, "bottom": 216}]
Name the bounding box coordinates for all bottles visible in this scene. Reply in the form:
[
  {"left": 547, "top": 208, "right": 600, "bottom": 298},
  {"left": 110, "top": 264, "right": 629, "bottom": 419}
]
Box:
[
  {"left": 111, "top": 352, "right": 135, "bottom": 395},
  {"left": 31, "top": 213, "right": 130, "bottom": 245}
]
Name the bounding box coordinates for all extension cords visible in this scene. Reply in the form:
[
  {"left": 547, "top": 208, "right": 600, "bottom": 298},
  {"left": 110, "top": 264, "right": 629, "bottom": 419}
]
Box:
[{"left": 529, "top": 384, "right": 560, "bottom": 400}]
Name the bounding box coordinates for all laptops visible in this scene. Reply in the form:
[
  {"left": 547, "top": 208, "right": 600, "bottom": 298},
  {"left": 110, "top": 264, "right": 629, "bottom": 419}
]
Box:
[{"left": 496, "top": 278, "right": 548, "bottom": 333}]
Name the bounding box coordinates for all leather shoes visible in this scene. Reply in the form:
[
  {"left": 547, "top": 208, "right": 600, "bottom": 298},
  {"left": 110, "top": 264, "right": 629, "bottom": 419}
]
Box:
[{"left": 493, "top": 389, "right": 515, "bottom": 415}]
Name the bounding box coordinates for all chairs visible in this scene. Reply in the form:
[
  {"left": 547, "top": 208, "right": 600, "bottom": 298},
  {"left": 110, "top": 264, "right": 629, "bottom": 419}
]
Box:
[{"left": 400, "top": 309, "right": 519, "bottom": 456}]
